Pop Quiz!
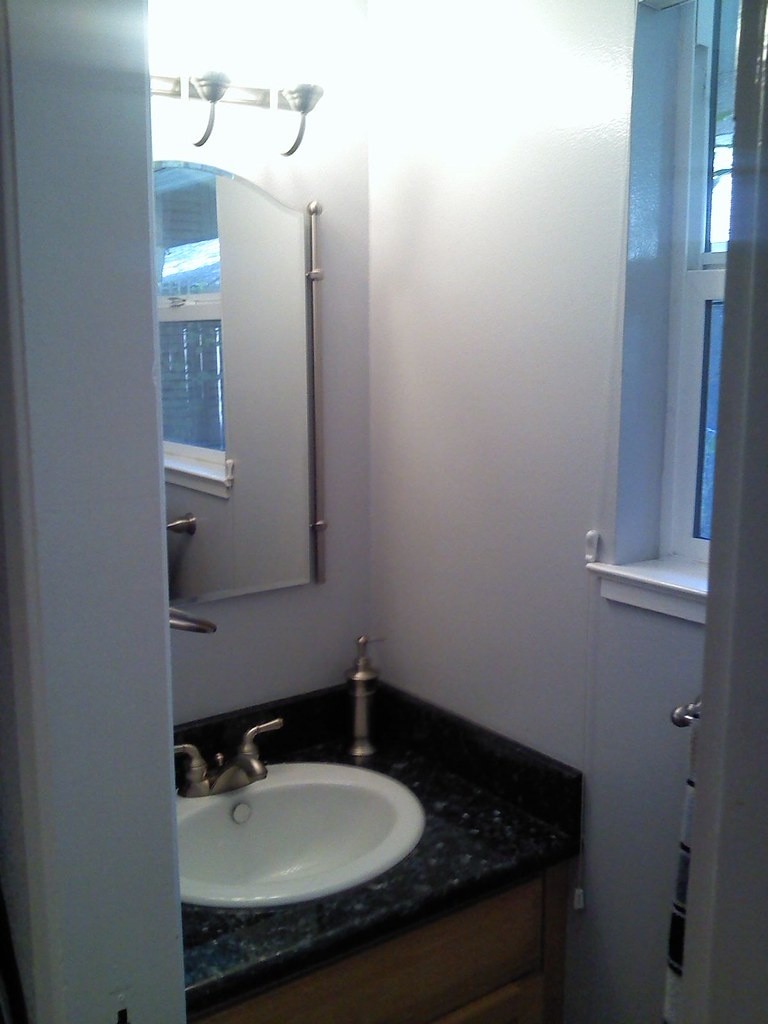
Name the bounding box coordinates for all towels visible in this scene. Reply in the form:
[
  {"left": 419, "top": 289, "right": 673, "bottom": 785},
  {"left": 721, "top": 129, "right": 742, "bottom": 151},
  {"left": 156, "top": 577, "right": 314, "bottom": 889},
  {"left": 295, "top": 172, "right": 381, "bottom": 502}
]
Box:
[{"left": 659, "top": 720, "right": 700, "bottom": 1023}]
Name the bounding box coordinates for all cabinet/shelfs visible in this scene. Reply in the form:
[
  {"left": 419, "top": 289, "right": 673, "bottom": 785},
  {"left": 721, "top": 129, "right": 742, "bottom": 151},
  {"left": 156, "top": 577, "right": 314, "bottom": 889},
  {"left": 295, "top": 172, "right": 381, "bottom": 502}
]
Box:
[{"left": 189, "top": 872, "right": 564, "bottom": 1024}]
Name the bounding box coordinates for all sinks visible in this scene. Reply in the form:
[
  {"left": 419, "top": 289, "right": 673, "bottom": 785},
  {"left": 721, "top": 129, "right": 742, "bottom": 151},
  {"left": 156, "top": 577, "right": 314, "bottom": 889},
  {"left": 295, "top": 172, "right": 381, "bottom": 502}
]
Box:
[{"left": 175, "top": 762, "right": 426, "bottom": 909}]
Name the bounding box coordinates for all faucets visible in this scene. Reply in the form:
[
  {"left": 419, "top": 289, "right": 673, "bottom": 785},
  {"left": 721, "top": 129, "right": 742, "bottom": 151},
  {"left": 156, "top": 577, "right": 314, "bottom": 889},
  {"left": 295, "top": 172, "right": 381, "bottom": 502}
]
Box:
[{"left": 173, "top": 717, "right": 287, "bottom": 798}]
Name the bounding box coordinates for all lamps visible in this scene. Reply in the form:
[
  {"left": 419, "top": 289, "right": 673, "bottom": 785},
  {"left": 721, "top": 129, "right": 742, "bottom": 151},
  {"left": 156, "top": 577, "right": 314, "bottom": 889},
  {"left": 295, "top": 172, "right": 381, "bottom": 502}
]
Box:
[
  {"left": 276, "top": 46, "right": 324, "bottom": 153},
  {"left": 185, "top": 24, "right": 240, "bottom": 146}
]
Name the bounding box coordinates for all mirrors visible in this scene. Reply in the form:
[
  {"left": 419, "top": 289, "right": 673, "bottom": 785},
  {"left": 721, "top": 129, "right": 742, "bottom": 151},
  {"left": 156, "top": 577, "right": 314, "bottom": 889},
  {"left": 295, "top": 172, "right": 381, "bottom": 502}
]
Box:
[{"left": 153, "top": 157, "right": 320, "bottom": 607}]
